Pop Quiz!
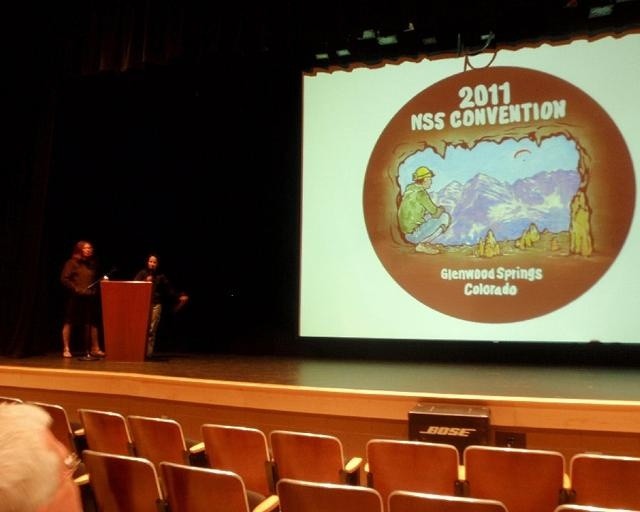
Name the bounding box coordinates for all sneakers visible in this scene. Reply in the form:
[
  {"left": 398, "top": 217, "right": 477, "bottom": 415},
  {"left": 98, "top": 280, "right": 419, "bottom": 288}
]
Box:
[
  {"left": 64, "top": 352, "right": 72, "bottom": 357},
  {"left": 91, "top": 350, "right": 105, "bottom": 357}
]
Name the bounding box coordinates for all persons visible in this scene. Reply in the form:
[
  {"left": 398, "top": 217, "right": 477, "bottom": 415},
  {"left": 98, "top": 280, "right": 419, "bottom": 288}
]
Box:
[
  {"left": 396, "top": 166, "right": 454, "bottom": 255},
  {"left": 135, "top": 254, "right": 188, "bottom": 361},
  {"left": 59, "top": 239, "right": 106, "bottom": 357},
  {"left": 0, "top": 401, "right": 83, "bottom": 512}
]
